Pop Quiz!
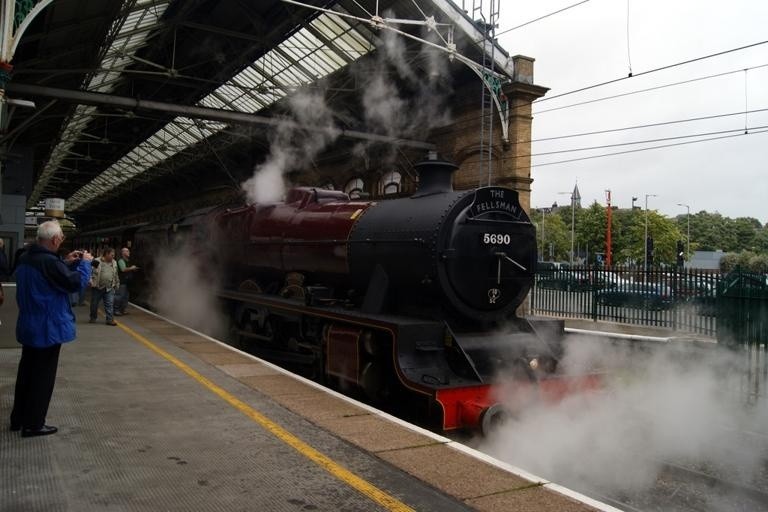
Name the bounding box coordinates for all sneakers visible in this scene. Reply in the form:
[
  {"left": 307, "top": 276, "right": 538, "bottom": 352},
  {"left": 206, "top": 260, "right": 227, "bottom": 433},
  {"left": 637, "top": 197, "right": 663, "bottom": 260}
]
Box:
[{"left": 88, "top": 310, "right": 129, "bottom": 326}]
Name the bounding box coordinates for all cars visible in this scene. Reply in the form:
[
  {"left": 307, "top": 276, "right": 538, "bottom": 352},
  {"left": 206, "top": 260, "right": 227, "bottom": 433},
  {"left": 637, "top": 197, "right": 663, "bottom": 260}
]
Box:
[{"left": 537, "top": 261, "right": 716, "bottom": 317}]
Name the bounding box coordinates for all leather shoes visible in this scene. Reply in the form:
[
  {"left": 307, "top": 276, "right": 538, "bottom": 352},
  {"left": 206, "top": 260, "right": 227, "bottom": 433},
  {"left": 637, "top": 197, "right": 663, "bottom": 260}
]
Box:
[
  {"left": 21, "top": 423, "right": 58, "bottom": 437},
  {"left": 9, "top": 422, "right": 21, "bottom": 432}
]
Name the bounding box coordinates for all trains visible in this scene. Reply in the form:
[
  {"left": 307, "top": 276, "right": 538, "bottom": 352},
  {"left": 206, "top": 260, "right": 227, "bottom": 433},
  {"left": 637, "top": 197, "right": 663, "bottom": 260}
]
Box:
[{"left": 72, "top": 160, "right": 615, "bottom": 437}]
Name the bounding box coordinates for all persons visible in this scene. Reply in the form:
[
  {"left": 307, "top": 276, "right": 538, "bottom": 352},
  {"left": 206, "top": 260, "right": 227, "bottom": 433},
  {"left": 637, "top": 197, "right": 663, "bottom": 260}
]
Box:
[
  {"left": 66, "top": 243, "right": 140, "bottom": 327},
  {"left": 9, "top": 220, "right": 95, "bottom": 438}
]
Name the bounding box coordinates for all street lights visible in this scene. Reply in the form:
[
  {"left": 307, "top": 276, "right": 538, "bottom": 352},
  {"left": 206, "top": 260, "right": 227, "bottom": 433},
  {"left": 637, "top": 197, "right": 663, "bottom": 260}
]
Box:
[
  {"left": 604, "top": 189, "right": 611, "bottom": 266},
  {"left": 644, "top": 194, "right": 657, "bottom": 283},
  {"left": 678, "top": 203, "right": 690, "bottom": 260},
  {"left": 632, "top": 196, "right": 637, "bottom": 208},
  {"left": 535, "top": 208, "right": 545, "bottom": 261},
  {"left": 557, "top": 191, "right": 575, "bottom": 270}
]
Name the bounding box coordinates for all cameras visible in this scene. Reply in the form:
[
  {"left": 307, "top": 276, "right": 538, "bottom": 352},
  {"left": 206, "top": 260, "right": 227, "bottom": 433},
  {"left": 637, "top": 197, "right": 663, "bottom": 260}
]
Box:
[{"left": 78, "top": 249, "right": 100, "bottom": 268}]
